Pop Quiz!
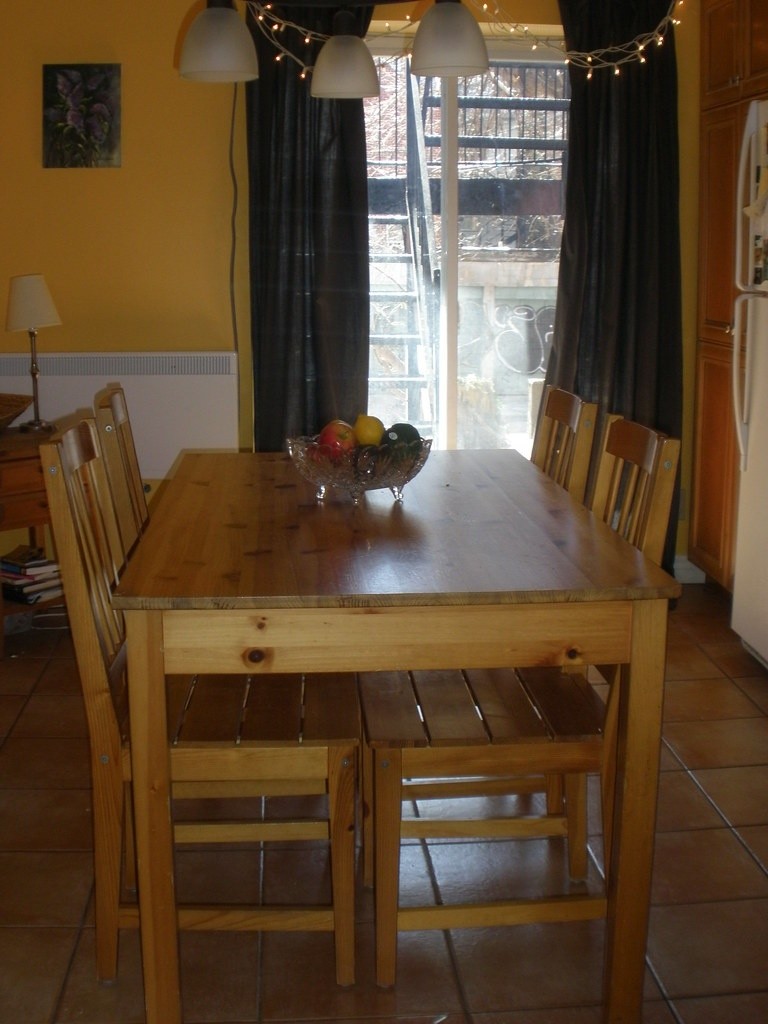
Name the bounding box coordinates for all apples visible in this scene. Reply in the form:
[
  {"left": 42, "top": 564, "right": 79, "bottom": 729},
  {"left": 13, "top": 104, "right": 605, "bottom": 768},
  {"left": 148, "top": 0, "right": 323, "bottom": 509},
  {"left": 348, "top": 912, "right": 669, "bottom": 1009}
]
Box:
[{"left": 317, "top": 419, "right": 355, "bottom": 463}]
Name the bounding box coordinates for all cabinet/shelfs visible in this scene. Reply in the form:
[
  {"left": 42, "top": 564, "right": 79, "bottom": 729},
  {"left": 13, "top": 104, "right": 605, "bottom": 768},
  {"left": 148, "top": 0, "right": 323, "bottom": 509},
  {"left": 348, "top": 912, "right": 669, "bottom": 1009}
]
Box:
[
  {"left": 687, "top": 0, "right": 768, "bottom": 593},
  {"left": 0, "top": 428, "right": 66, "bottom": 659}
]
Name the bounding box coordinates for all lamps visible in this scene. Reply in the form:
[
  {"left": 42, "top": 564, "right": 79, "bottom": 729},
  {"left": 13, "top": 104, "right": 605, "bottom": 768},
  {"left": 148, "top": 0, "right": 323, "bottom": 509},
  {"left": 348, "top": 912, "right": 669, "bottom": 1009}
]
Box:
[
  {"left": 411, "top": 0, "right": 489, "bottom": 80},
  {"left": 309, "top": 0, "right": 381, "bottom": 100},
  {"left": 178, "top": 0, "right": 259, "bottom": 84},
  {"left": 5, "top": 272, "right": 63, "bottom": 434}
]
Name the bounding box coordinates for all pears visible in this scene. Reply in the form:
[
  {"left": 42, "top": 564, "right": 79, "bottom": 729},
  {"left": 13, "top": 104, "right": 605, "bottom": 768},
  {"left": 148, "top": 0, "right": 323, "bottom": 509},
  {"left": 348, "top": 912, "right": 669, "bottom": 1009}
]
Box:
[{"left": 353, "top": 411, "right": 385, "bottom": 446}]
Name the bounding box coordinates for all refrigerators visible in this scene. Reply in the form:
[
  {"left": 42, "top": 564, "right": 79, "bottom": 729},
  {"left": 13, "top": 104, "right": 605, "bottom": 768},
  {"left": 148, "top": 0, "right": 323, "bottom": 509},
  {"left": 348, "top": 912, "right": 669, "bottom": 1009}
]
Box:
[{"left": 730, "top": 100, "right": 768, "bottom": 666}]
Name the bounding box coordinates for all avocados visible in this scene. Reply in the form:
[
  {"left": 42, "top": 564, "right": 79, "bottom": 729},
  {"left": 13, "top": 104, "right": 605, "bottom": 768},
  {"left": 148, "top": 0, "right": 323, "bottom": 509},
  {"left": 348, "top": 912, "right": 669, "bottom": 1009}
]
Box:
[{"left": 350, "top": 422, "right": 422, "bottom": 478}]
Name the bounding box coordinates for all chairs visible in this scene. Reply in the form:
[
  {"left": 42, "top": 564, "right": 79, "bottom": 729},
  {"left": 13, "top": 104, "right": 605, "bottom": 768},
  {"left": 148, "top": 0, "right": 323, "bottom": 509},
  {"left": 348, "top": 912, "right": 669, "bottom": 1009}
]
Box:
[{"left": 38, "top": 384, "right": 684, "bottom": 989}]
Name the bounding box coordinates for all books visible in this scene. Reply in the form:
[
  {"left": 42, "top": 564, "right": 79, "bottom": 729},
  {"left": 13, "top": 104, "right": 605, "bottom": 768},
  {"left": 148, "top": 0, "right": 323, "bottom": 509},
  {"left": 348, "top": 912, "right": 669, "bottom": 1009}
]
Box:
[{"left": 0, "top": 544, "right": 64, "bottom": 604}]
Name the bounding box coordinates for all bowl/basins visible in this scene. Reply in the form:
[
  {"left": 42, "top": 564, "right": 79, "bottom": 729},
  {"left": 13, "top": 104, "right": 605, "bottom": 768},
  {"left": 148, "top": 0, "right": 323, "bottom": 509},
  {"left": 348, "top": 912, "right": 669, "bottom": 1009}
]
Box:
[{"left": 287, "top": 435, "right": 433, "bottom": 505}]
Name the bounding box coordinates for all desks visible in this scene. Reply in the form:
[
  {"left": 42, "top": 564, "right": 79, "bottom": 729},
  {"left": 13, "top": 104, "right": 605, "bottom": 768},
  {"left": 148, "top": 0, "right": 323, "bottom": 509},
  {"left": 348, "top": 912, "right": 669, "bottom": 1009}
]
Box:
[{"left": 110, "top": 449, "right": 684, "bottom": 1024}]
